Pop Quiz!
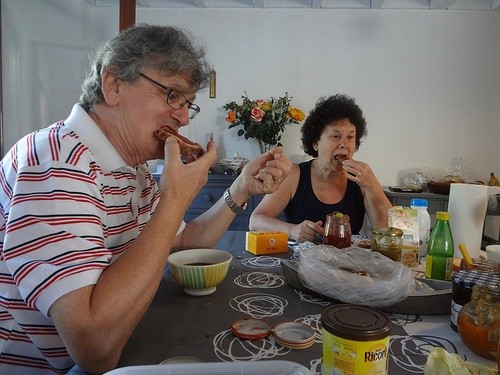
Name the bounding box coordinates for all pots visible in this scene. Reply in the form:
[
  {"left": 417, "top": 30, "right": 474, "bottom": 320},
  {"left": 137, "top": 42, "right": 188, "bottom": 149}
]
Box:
[{"left": 219, "top": 152, "right": 249, "bottom": 174}]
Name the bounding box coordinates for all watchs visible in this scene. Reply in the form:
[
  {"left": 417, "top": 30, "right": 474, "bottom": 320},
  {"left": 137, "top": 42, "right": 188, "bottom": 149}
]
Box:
[{"left": 223, "top": 187, "right": 247, "bottom": 215}]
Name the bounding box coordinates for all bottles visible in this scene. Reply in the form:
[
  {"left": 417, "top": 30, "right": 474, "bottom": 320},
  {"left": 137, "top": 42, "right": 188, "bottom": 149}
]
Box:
[
  {"left": 370, "top": 226, "right": 404, "bottom": 262},
  {"left": 322, "top": 214, "right": 352, "bottom": 249},
  {"left": 425, "top": 211, "right": 455, "bottom": 281},
  {"left": 410, "top": 198, "right": 431, "bottom": 257}
]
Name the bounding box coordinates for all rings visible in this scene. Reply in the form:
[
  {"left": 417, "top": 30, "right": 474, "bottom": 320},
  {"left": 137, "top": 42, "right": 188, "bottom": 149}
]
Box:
[{"left": 275, "top": 178, "right": 283, "bottom": 182}]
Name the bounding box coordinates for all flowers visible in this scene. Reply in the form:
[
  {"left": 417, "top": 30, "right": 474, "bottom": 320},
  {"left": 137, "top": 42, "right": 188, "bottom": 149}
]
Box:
[{"left": 223, "top": 90, "right": 305, "bottom": 152}]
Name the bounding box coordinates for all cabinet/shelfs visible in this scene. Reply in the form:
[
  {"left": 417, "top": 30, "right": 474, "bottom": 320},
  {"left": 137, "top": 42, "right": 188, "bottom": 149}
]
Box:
[
  {"left": 153, "top": 174, "right": 296, "bottom": 229},
  {"left": 383, "top": 188, "right": 500, "bottom": 230}
]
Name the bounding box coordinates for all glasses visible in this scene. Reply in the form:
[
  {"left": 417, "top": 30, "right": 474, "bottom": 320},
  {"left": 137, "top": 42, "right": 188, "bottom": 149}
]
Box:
[{"left": 139, "top": 70, "right": 200, "bottom": 119}]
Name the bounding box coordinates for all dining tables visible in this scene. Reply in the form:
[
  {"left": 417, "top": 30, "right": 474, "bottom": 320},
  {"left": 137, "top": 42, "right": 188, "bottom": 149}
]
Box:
[{"left": 78, "top": 230, "right": 500, "bottom": 375}]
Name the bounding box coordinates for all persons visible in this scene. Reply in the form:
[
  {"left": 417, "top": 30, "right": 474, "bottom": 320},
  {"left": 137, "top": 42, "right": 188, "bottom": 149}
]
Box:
[
  {"left": 0, "top": 22, "right": 293, "bottom": 375},
  {"left": 249, "top": 93, "right": 392, "bottom": 243}
]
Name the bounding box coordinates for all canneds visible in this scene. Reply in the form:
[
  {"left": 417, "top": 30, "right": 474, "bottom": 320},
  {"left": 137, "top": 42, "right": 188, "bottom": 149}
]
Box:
[
  {"left": 324, "top": 213, "right": 352, "bottom": 249},
  {"left": 321, "top": 304, "right": 391, "bottom": 375},
  {"left": 457, "top": 285, "right": 499, "bottom": 361},
  {"left": 451, "top": 258, "right": 500, "bottom": 332},
  {"left": 371, "top": 227, "right": 404, "bottom": 262}
]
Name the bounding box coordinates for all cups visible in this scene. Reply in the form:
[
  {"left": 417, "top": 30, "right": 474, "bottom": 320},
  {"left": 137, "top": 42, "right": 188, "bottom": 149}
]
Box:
[
  {"left": 485, "top": 244, "right": 500, "bottom": 264},
  {"left": 448, "top": 157, "right": 464, "bottom": 177}
]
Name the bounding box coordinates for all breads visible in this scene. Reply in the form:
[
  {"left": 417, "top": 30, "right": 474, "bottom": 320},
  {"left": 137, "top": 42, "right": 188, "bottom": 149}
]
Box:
[
  {"left": 156, "top": 129, "right": 204, "bottom": 164},
  {"left": 338, "top": 156, "right": 352, "bottom": 163}
]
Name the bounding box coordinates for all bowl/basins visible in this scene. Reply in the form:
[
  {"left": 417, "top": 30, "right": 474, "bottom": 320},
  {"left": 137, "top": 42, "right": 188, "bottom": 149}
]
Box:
[{"left": 167, "top": 248, "right": 233, "bottom": 296}]
